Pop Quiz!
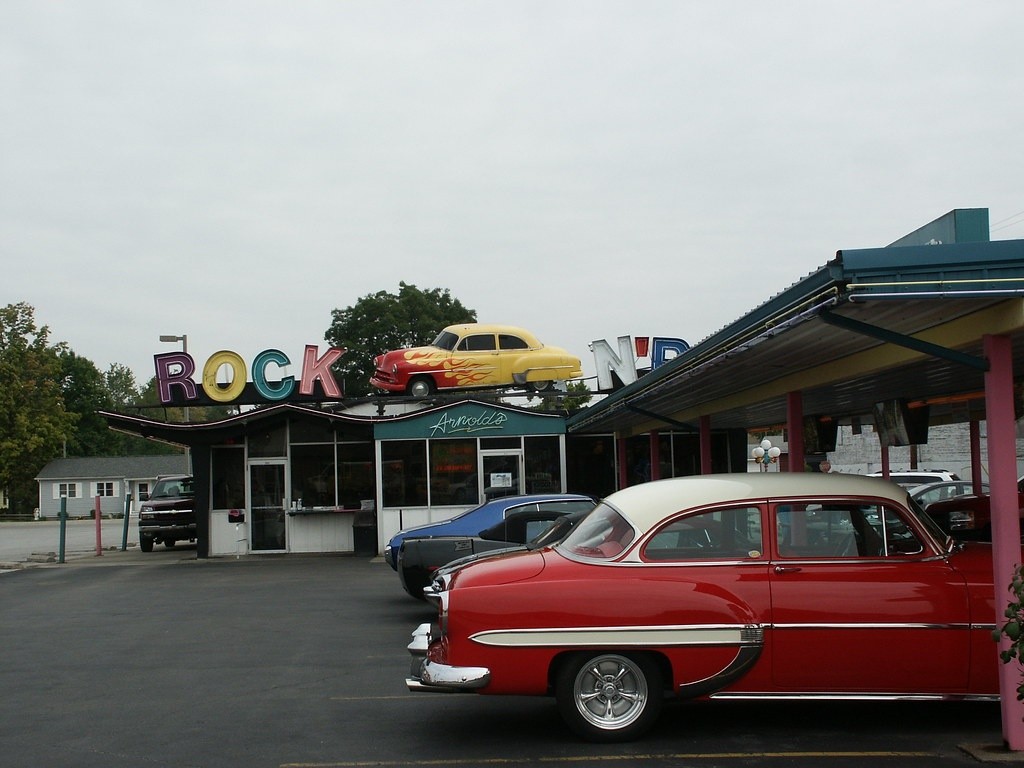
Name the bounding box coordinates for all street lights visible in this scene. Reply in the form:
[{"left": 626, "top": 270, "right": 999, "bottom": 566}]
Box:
[
  {"left": 753, "top": 439, "right": 780, "bottom": 471},
  {"left": 159, "top": 335, "right": 189, "bottom": 356}
]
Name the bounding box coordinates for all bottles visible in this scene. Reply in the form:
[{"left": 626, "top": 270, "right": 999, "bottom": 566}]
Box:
[{"left": 297, "top": 498, "right": 303, "bottom": 510}]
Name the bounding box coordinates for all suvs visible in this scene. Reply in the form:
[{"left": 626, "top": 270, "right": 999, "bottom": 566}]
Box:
[{"left": 138, "top": 474, "right": 198, "bottom": 554}]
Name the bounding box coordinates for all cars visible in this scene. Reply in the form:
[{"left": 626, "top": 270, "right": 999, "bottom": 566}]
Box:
[
  {"left": 385, "top": 493, "right": 751, "bottom": 611},
  {"left": 781, "top": 469, "right": 1024, "bottom": 545},
  {"left": 406, "top": 472, "right": 1002, "bottom": 740},
  {"left": 370, "top": 324, "right": 582, "bottom": 399}
]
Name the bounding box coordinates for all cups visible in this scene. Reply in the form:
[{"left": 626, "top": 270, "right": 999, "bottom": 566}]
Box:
[{"left": 292, "top": 502, "right": 296, "bottom": 509}]
